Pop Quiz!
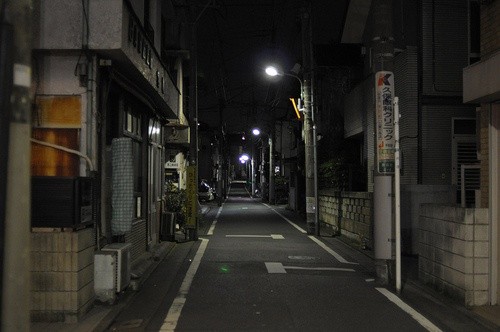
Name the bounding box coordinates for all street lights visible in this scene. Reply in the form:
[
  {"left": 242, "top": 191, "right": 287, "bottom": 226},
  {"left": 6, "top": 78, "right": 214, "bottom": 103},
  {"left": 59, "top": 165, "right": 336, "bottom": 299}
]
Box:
[
  {"left": 264, "top": 65, "right": 320, "bottom": 236},
  {"left": 251, "top": 128, "right": 275, "bottom": 204}
]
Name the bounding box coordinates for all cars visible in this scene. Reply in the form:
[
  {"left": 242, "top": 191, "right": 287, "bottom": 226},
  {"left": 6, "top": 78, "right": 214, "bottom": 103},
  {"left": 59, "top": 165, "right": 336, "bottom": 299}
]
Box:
[{"left": 198, "top": 181, "right": 213, "bottom": 201}]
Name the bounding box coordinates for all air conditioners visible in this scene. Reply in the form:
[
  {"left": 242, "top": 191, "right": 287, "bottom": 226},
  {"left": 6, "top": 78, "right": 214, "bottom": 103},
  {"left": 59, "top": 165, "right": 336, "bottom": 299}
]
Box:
[
  {"left": 161, "top": 212, "right": 176, "bottom": 236},
  {"left": 102, "top": 243, "right": 130, "bottom": 292}
]
